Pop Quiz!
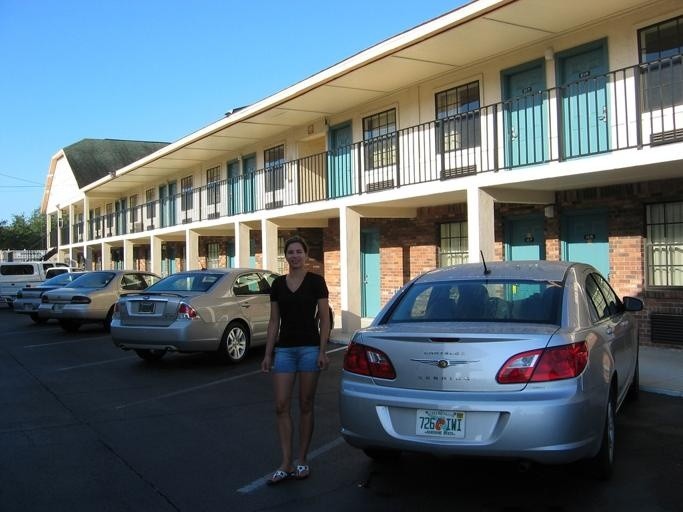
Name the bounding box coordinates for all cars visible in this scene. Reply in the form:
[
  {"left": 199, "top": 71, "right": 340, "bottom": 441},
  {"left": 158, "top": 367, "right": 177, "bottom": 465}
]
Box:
[{"left": 340, "top": 250, "right": 644, "bottom": 483}]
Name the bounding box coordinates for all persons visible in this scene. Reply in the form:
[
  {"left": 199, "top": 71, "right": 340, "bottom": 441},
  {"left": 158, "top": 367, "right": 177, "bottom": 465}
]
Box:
[{"left": 261, "top": 235, "right": 331, "bottom": 485}]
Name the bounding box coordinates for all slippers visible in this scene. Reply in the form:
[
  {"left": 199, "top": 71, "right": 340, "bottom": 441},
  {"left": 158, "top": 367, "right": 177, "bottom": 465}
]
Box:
[
  {"left": 268, "top": 470, "right": 295, "bottom": 484},
  {"left": 296, "top": 464, "right": 309, "bottom": 478}
]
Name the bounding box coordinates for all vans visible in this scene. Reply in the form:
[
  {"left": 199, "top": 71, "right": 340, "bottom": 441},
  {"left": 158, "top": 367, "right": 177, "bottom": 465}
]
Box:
[{"left": 0, "top": 261, "right": 84, "bottom": 307}]
